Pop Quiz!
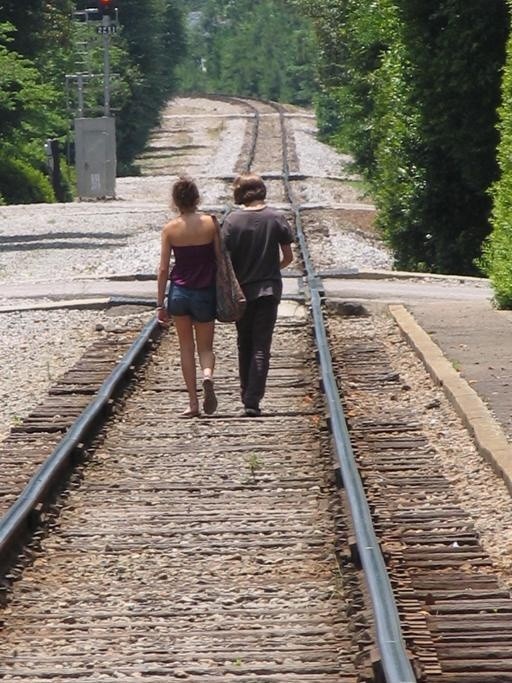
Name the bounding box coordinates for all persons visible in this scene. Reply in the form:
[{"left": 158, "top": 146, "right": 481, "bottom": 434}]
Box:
[
  {"left": 156, "top": 180, "right": 221, "bottom": 418},
  {"left": 220, "top": 174, "right": 293, "bottom": 415}
]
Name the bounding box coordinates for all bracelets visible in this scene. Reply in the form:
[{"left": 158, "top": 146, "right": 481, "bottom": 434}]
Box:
[{"left": 156, "top": 305, "right": 165, "bottom": 310}]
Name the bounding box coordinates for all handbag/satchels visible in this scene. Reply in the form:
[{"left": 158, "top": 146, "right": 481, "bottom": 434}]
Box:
[{"left": 216, "top": 251, "right": 247, "bottom": 322}]
